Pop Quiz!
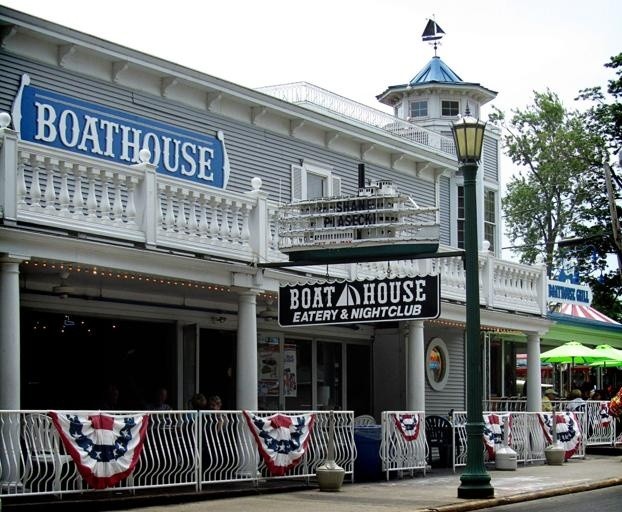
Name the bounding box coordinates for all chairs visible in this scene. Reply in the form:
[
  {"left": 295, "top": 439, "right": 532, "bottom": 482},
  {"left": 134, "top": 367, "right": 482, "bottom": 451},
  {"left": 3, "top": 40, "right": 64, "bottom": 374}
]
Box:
[
  {"left": 348, "top": 415, "right": 376, "bottom": 426},
  {"left": 24, "top": 414, "right": 81, "bottom": 491},
  {"left": 426, "top": 416, "right": 460, "bottom": 468}
]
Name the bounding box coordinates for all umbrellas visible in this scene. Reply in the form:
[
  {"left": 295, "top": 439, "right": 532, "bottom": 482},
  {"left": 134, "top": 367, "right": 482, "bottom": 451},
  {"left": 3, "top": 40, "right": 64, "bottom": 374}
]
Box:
[
  {"left": 538, "top": 340, "right": 612, "bottom": 391},
  {"left": 585, "top": 344, "right": 622, "bottom": 389}
]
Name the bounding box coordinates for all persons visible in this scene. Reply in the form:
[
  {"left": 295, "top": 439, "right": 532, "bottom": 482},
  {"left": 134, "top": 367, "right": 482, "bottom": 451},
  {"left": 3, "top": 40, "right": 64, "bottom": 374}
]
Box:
[
  {"left": 540, "top": 387, "right": 558, "bottom": 412},
  {"left": 94, "top": 383, "right": 225, "bottom": 411},
  {"left": 556, "top": 379, "right": 622, "bottom": 442}
]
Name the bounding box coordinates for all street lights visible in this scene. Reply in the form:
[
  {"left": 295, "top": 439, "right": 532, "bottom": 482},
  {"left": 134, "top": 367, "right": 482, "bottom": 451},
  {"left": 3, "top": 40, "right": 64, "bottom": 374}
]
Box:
[{"left": 450, "top": 102, "right": 497, "bottom": 501}]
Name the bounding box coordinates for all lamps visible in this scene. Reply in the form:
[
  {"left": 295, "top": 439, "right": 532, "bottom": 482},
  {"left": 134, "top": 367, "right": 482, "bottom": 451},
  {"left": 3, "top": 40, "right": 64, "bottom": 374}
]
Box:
[
  {"left": 261, "top": 299, "right": 277, "bottom": 321},
  {"left": 53, "top": 270, "right": 73, "bottom": 299}
]
